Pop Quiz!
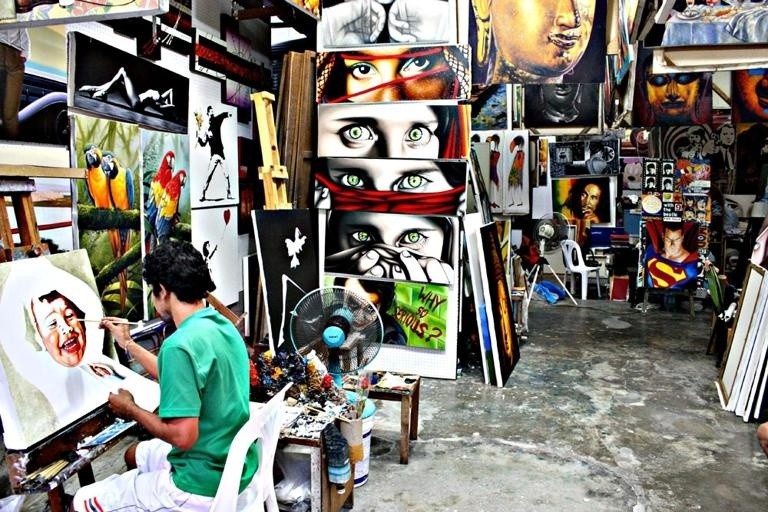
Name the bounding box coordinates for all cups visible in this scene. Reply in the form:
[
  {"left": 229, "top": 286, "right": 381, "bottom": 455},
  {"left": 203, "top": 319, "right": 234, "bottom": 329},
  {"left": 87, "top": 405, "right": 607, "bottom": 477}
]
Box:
[{"left": 340, "top": 418, "right": 363, "bottom": 464}]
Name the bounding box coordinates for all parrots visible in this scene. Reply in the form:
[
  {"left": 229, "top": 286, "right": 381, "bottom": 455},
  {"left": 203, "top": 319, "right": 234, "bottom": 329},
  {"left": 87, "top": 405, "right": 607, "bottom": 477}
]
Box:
[
  {"left": 146, "top": 150, "right": 176, "bottom": 241},
  {"left": 100, "top": 150, "right": 135, "bottom": 314},
  {"left": 83, "top": 142, "right": 127, "bottom": 292},
  {"left": 155, "top": 169, "right": 187, "bottom": 247}
]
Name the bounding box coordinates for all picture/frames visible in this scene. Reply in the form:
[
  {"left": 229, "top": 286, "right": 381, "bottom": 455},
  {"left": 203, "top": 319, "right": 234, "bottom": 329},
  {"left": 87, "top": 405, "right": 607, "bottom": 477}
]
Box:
[{"left": 714, "top": 262, "right": 768, "bottom": 425}]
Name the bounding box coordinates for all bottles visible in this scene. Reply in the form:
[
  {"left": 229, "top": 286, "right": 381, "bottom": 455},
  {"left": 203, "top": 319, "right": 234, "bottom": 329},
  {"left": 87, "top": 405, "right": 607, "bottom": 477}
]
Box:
[{"left": 325, "top": 436, "right": 352, "bottom": 495}]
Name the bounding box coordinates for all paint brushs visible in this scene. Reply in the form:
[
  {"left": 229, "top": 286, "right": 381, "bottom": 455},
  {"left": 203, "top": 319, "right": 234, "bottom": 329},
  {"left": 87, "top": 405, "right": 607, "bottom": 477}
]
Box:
[
  {"left": 305, "top": 406, "right": 350, "bottom": 423},
  {"left": 17, "top": 459, "right": 70, "bottom": 490},
  {"left": 77, "top": 421, "right": 116, "bottom": 451},
  {"left": 69, "top": 319, "right": 143, "bottom": 324}
]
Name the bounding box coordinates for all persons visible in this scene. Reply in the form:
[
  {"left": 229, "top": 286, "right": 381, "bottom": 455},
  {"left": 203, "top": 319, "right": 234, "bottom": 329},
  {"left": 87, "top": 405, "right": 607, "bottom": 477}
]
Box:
[
  {"left": 704, "top": 126, "right": 737, "bottom": 169},
  {"left": 674, "top": 125, "right": 702, "bottom": 162},
  {"left": 199, "top": 234, "right": 220, "bottom": 309},
  {"left": 0, "top": 16, "right": 33, "bottom": 137},
  {"left": 70, "top": 239, "right": 260, "bottom": 512},
  {"left": 642, "top": 68, "right": 710, "bottom": 125},
  {"left": 78, "top": 62, "right": 179, "bottom": 115},
  {"left": 29, "top": 289, "right": 89, "bottom": 369},
  {"left": 193, "top": 99, "right": 239, "bottom": 206},
  {"left": 528, "top": 80, "right": 591, "bottom": 126},
  {"left": 470, "top": 1, "right": 601, "bottom": 83},
  {"left": 558, "top": 158, "right": 755, "bottom": 295}
]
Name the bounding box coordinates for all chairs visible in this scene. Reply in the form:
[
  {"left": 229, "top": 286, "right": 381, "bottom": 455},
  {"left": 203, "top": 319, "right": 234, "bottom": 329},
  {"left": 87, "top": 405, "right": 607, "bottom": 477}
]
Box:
[
  {"left": 556, "top": 238, "right": 603, "bottom": 300},
  {"left": 214, "top": 378, "right": 296, "bottom": 512}
]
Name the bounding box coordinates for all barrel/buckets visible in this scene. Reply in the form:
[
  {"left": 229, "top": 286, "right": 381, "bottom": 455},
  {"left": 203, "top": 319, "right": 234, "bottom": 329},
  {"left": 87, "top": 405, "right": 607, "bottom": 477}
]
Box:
[
  {"left": 623, "top": 209, "right": 640, "bottom": 236},
  {"left": 344, "top": 391, "right": 375, "bottom": 488}
]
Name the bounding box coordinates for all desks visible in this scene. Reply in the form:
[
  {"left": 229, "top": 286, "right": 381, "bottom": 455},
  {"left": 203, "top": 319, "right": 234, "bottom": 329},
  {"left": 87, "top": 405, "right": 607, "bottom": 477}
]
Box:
[
  {"left": 274, "top": 389, "right": 357, "bottom": 512},
  {"left": 340, "top": 371, "right": 427, "bottom": 466}
]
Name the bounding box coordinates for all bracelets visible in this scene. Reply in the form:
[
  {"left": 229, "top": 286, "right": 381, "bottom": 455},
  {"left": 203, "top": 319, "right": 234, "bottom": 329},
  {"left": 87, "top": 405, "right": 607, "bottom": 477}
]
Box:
[{"left": 123, "top": 338, "right": 134, "bottom": 358}]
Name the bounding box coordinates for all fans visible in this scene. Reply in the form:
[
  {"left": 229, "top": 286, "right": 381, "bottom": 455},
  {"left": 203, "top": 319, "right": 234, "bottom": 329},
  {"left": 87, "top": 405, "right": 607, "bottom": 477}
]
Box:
[
  {"left": 288, "top": 285, "right": 386, "bottom": 420},
  {"left": 516, "top": 212, "right": 579, "bottom": 322}
]
